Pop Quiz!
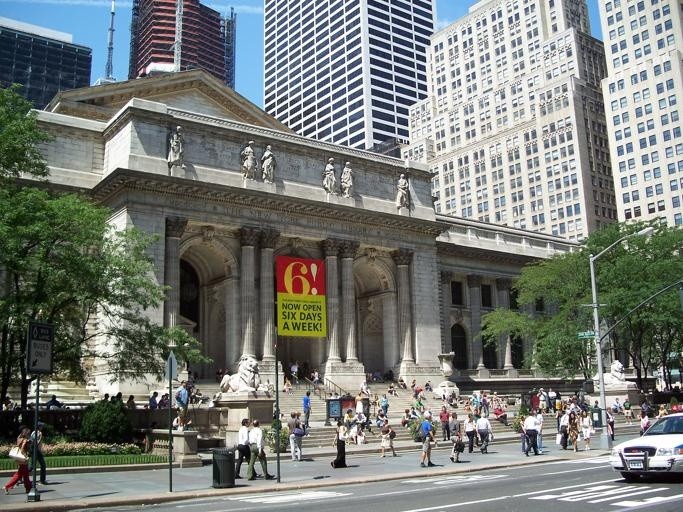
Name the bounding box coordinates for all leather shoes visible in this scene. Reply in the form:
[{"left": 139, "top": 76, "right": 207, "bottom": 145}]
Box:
[
  {"left": 248, "top": 474, "right": 275, "bottom": 480},
  {"left": 235, "top": 475, "right": 241, "bottom": 478}
]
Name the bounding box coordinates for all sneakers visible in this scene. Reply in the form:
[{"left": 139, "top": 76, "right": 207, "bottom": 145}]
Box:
[
  {"left": 450, "top": 457, "right": 461, "bottom": 463},
  {"left": 522, "top": 449, "right": 544, "bottom": 456},
  {"left": 420, "top": 462, "right": 434, "bottom": 466}
]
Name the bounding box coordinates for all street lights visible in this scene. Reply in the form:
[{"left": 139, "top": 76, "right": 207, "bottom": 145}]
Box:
[{"left": 588, "top": 227, "right": 655, "bottom": 452}]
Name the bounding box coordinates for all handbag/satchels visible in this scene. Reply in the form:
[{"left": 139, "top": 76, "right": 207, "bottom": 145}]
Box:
[
  {"left": 339, "top": 432, "right": 349, "bottom": 441},
  {"left": 293, "top": 428, "right": 303, "bottom": 437},
  {"left": 9, "top": 445, "right": 29, "bottom": 462},
  {"left": 389, "top": 431, "right": 396, "bottom": 439},
  {"left": 454, "top": 440, "right": 465, "bottom": 453}
]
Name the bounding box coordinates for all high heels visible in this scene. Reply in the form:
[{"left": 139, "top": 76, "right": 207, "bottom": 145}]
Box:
[{"left": 2, "top": 487, "right": 9, "bottom": 495}]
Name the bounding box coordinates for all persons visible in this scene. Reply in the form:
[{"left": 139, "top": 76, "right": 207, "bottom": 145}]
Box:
[
  {"left": 167, "top": 125, "right": 188, "bottom": 170},
  {"left": 396, "top": 173, "right": 409, "bottom": 209},
  {"left": 519, "top": 387, "right": 594, "bottom": 456},
  {"left": 328, "top": 379, "right": 398, "bottom": 457},
  {"left": 47, "top": 394, "right": 62, "bottom": 410},
  {"left": 340, "top": 161, "right": 355, "bottom": 198},
  {"left": 240, "top": 140, "right": 259, "bottom": 183},
  {"left": 1, "top": 425, "right": 49, "bottom": 496},
  {"left": 441, "top": 389, "right": 510, "bottom": 427},
  {"left": 260, "top": 144, "right": 277, "bottom": 183},
  {"left": 372, "top": 369, "right": 433, "bottom": 402},
  {"left": 593, "top": 398, "right": 668, "bottom": 441},
  {"left": 103, "top": 392, "right": 137, "bottom": 409},
  {"left": 322, "top": 157, "right": 339, "bottom": 195},
  {"left": 148, "top": 366, "right": 232, "bottom": 431},
  {"left": 304, "top": 391, "right": 312, "bottom": 429},
  {"left": 282, "top": 362, "right": 319, "bottom": 394},
  {"left": 235, "top": 404, "right": 304, "bottom": 480},
  {"left": 402, "top": 398, "right": 496, "bottom": 467}
]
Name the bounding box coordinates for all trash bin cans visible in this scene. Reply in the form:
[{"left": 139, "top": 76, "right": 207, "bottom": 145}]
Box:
[
  {"left": 213, "top": 449, "right": 235, "bottom": 489},
  {"left": 592, "top": 408, "right": 601, "bottom": 427}
]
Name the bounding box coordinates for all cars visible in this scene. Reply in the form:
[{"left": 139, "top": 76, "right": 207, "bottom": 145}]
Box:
[{"left": 608, "top": 404, "right": 683, "bottom": 482}]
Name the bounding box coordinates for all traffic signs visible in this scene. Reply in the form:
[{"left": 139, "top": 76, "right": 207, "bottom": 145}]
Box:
[
  {"left": 26, "top": 320, "right": 55, "bottom": 374},
  {"left": 576, "top": 331, "right": 595, "bottom": 339}
]
